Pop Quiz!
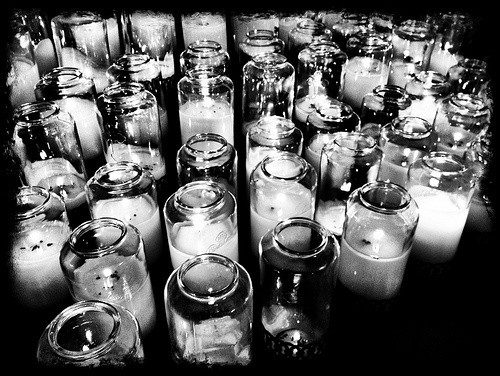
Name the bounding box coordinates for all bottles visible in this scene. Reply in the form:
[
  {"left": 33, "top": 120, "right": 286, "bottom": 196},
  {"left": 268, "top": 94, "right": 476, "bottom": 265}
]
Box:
[
  {"left": 6, "top": 8, "right": 495, "bottom": 308},
  {"left": 164, "top": 253, "right": 254, "bottom": 369},
  {"left": 36, "top": 301, "right": 144, "bottom": 370},
  {"left": 258, "top": 217, "right": 340, "bottom": 359},
  {"left": 59, "top": 216, "right": 155, "bottom": 338}
]
